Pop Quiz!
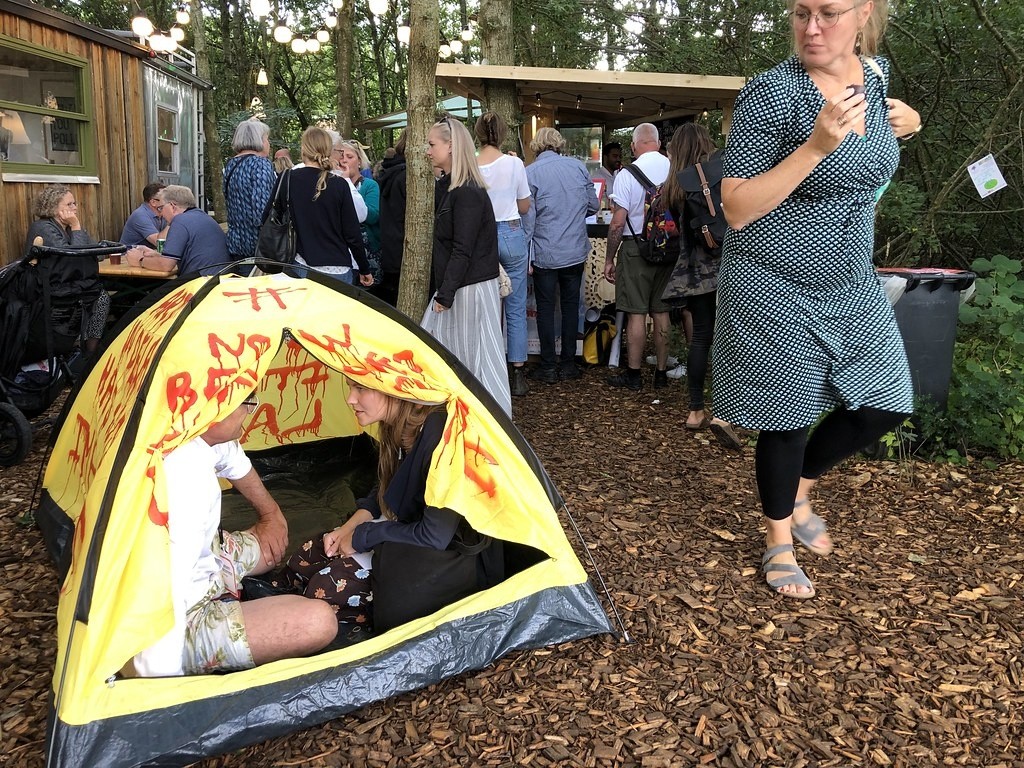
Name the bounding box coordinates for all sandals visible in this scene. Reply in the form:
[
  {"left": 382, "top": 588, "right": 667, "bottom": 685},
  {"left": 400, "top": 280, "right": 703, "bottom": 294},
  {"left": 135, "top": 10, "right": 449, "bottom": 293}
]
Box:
[{"left": 761, "top": 496, "right": 833, "bottom": 599}]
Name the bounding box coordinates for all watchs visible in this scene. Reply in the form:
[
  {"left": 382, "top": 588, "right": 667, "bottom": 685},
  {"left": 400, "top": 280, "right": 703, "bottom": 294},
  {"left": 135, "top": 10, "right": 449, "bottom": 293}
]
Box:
[
  {"left": 900, "top": 115, "right": 922, "bottom": 141},
  {"left": 140, "top": 257, "right": 144, "bottom": 268}
]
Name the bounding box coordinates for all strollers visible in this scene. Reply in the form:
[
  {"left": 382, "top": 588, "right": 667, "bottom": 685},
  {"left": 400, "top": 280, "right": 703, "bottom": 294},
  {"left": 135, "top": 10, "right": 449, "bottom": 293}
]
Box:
[{"left": 0, "top": 240, "right": 126, "bottom": 466}]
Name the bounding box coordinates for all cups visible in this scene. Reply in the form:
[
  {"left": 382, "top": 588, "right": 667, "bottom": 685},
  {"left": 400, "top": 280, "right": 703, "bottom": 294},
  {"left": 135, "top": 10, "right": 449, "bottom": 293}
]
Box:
[
  {"left": 584, "top": 307, "right": 601, "bottom": 323},
  {"left": 156, "top": 239, "right": 165, "bottom": 253},
  {"left": 110, "top": 253, "right": 121, "bottom": 264}
]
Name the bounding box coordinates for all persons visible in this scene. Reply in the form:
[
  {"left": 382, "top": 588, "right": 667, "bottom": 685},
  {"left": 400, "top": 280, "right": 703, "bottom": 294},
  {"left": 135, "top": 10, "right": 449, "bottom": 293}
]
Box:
[
  {"left": 117, "top": 391, "right": 339, "bottom": 676},
  {"left": 118, "top": 111, "right": 740, "bottom": 449},
  {"left": 267, "top": 375, "right": 505, "bottom": 636},
  {"left": 713, "top": 0, "right": 921, "bottom": 600},
  {"left": 25, "top": 184, "right": 110, "bottom": 359}
]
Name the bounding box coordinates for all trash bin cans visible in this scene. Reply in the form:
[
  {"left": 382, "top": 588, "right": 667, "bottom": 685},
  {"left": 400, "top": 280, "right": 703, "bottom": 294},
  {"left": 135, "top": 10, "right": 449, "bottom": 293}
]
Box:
[{"left": 864, "top": 267, "right": 976, "bottom": 459}]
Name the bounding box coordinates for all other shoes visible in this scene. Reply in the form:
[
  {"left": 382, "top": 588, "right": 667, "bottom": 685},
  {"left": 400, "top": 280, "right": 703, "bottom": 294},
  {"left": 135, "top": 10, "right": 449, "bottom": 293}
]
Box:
[
  {"left": 539, "top": 362, "right": 582, "bottom": 383},
  {"left": 606, "top": 371, "right": 644, "bottom": 390},
  {"left": 646, "top": 354, "right": 679, "bottom": 367},
  {"left": 666, "top": 364, "right": 688, "bottom": 379},
  {"left": 654, "top": 367, "right": 668, "bottom": 389},
  {"left": 512, "top": 368, "right": 531, "bottom": 396}
]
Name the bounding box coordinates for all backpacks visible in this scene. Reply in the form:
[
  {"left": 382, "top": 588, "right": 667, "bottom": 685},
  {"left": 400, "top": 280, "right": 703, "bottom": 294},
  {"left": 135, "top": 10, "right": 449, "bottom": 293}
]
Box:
[
  {"left": 618, "top": 164, "right": 677, "bottom": 264},
  {"left": 676, "top": 159, "right": 727, "bottom": 256}
]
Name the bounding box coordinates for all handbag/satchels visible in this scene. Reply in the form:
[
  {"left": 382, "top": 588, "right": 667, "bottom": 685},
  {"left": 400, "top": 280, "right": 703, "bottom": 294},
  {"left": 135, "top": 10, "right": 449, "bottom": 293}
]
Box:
[
  {"left": 582, "top": 317, "right": 618, "bottom": 366},
  {"left": 255, "top": 168, "right": 298, "bottom": 273}
]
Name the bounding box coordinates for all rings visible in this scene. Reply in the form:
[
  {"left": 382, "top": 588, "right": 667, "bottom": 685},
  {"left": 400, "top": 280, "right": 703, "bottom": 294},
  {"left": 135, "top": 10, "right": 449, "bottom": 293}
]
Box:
[{"left": 837, "top": 116, "right": 846, "bottom": 125}]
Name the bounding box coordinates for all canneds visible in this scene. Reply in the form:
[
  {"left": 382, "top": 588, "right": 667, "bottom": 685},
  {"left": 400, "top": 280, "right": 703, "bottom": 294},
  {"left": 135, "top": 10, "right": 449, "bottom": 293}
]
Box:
[{"left": 156, "top": 239, "right": 165, "bottom": 253}]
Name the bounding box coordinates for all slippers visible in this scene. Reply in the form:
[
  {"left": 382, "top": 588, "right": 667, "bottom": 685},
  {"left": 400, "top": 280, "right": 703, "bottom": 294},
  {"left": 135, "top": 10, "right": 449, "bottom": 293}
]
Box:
[
  {"left": 685, "top": 410, "right": 707, "bottom": 430},
  {"left": 709, "top": 418, "right": 740, "bottom": 448}
]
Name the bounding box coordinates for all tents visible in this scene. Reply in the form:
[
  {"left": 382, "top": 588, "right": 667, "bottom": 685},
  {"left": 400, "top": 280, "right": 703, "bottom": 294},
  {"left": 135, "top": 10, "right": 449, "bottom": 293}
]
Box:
[{"left": 28, "top": 258, "right": 631, "bottom": 768}]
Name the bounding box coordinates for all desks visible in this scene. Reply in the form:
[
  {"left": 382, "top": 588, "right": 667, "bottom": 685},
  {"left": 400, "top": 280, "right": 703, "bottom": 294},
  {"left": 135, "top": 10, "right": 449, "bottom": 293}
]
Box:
[{"left": 79, "top": 245, "right": 177, "bottom": 278}]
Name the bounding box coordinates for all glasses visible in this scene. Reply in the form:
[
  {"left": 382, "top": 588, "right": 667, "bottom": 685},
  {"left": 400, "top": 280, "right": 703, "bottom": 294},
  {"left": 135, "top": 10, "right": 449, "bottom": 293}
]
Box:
[
  {"left": 334, "top": 149, "right": 344, "bottom": 156},
  {"left": 787, "top": 5, "right": 857, "bottom": 32},
  {"left": 156, "top": 205, "right": 165, "bottom": 212},
  {"left": 241, "top": 394, "right": 260, "bottom": 415},
  {"left": 439, "top": 116, "right": 452, "bottom": 132}
]
